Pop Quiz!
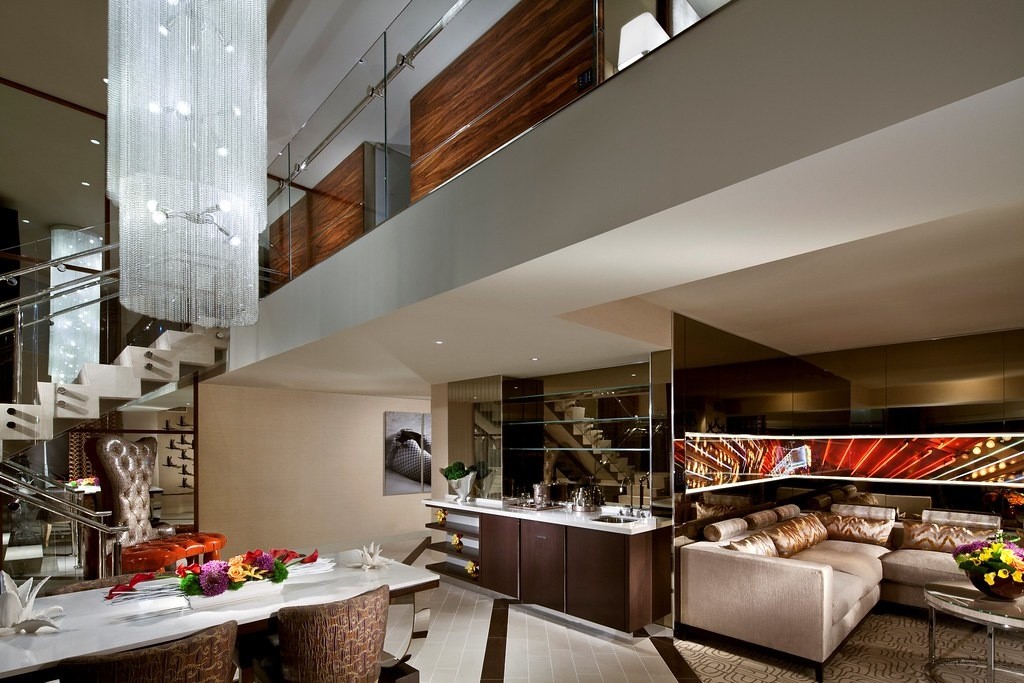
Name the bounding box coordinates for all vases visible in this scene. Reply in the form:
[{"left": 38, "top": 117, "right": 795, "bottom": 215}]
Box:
[
  {"left": 471, "top": 561, "right": 479, "bottom": 581},
  {"left": 440, "top": 509, "right": 446, "bottom": 526},
  {"left": 965, "top": 569, "right": 1024, "bottom": 600},
  {"left": 456, "top": 534, "right": 463, "bottom": 551},
  {"left": 184, "top": 579, "right": 285, "bottom": 610}
]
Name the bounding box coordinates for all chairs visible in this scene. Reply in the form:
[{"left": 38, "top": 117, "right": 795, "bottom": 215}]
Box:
[
  {"left": 277, "top": 584, "right": 390, "bottom": 683},
  {"left": 95, "top": 435, "right": 228, "bottom": 572},
  {"left": 56, "top": 620, "right": 237, "bottom": 683},
  {"left": 52, "top": 572, "right": 158, "bottom": 595},
  {"left": 40, "top": 489, "right": 77, "bottom": 547}
]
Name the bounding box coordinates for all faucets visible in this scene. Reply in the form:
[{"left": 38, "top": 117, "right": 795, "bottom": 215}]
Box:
[
  {"left": 619, "top": 476, "right": 634, "bottom": 517},
  {"left": 638, "top": 476, "right": 650, "bottom": 510}
]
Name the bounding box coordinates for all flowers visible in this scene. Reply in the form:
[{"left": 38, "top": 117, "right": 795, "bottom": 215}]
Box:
[
  {"left": 953, "top": 530, "right": 1024, "bottom": 585},
  {"left": 105, "top": 549, "right": 320, "bottom": 596},
  {"left": 465, "top": 561, "right": 475, "bottom": 574},
  {"left": 451, "top": 534, "right": 459, "bottom": 546},
  {"left": 432, "top": 509, "right": 444, "bottom": 524}
]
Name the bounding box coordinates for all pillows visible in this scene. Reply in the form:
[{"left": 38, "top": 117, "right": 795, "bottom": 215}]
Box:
[
  {"left": 767, "top": 513, "right": 828, "bottom": 557},
  {"left": 816, "top": 513, "right": 896, "bottom": 549},
  {"left": 903, "top": 520, "right": 1001, "bottom": 555},
  {"left": 730, "top": 532, "right": 779, "bottom": 557}
]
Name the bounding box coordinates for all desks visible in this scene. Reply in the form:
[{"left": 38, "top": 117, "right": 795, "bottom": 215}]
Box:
[
  {"left": 65, "top": 482, "right": 163, "bottom": 521},
  {"left": 0, "top": 548, "right": 440, "bottom": 683}
]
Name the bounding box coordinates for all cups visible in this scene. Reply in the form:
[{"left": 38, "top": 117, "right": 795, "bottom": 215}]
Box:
[{"left": 522, "top": 492, "right": 530, "bottom": 508}]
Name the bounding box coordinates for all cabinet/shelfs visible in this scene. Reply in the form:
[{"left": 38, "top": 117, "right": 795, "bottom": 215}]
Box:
[
  {"left": 519, "top": 519, "right": 565, "bottom": 616},
  {"left": 507, "top": 382, "right": 652, "bottom": 454},
  {"left": 480, "top": 514, "right": 519, "bottom": 600},
  {"left": 565, "top": 527, "right": 653, "bottom": 635},
  {"left": 423, "top": 505, "right": 482, "bottom": 588}
]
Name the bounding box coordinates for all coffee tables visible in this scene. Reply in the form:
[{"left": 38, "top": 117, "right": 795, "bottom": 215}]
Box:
[{"left": 922, "top": 580, "right": 1024, "bottom": 683}]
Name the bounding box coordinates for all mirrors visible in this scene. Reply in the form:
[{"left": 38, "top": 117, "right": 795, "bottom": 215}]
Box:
[
  {"left": 508, "top": 349, "right": 650, "bottom": 515},
  {"left": 445, "top": 377, "right": 504, "bottom": 500}
]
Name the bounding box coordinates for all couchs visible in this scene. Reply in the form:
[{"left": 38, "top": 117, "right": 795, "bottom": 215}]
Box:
[
  {"left": 881, "top": 509, "right": 1003, "bottom": 611},
  {"left": 679, "top": 503, "right": 903, "bottom": 682}
]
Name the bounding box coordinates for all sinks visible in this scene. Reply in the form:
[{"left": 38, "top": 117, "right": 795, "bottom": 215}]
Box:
[{"left": 589, "top": 515, "right": 638, "bottom": 523}]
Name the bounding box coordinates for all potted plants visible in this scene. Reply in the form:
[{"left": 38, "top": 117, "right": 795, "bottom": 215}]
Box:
[
  {"left": 443, "top": 462, "right": 479, "bottom": 502},
  {"left": 468, "top": 461, "right": 496, "bottom": 499}
]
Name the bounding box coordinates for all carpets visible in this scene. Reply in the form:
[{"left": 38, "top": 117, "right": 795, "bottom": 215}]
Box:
[{"left": 674, "top": 612, "right": 1024, "bottom": 683}]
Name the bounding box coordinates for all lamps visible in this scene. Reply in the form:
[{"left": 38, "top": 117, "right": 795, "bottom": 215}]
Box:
[
  {"left": 107, "top": 0, "right": 267, "bottom": 328},
  {"left": 47, "top": 229, "right": 102, "bottom": 385}
]
